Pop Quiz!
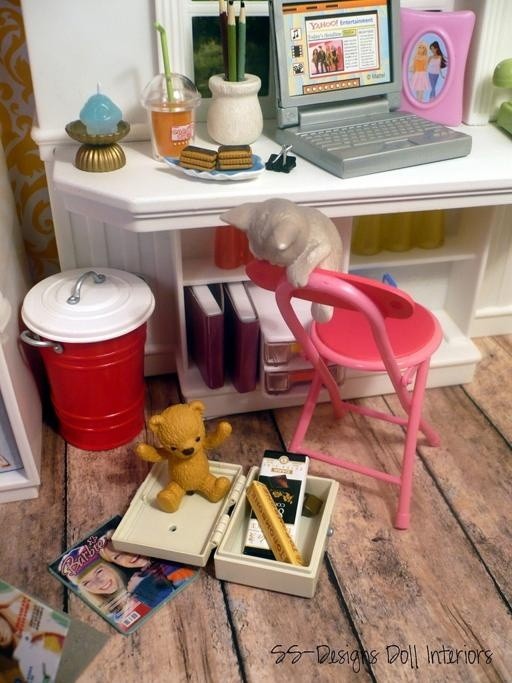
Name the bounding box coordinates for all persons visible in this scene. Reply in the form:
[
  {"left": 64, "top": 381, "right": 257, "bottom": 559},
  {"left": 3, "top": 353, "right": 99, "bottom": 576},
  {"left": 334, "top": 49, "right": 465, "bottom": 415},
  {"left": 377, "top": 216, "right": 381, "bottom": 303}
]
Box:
[
  {"left": 409, "top": 41, "right": 431, "bottom": 102},
  {"left": 58, "top": 547, "right": 147, "bottom": 628},
  {"left": 312, "top": 46, "right": 342, "bottom": 73},
  {"left": 0, "top": 602, "right": 31, "bottom": 653},
  {"left": 95, "top": 531, "right": 193, "bottom": 609},
  {"left": 426, "top": 40, "right": 446, "bottom": 99}
]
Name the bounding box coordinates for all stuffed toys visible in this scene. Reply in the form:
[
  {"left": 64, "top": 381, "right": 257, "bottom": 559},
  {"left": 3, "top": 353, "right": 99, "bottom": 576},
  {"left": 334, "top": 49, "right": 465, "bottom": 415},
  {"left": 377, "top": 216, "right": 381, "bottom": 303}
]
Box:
[
  {"left": 133, "top": 399, "right": 233, "bottom": 511},
  {"left": 220, "top": 197, "right": 344, "bottom": 323}
]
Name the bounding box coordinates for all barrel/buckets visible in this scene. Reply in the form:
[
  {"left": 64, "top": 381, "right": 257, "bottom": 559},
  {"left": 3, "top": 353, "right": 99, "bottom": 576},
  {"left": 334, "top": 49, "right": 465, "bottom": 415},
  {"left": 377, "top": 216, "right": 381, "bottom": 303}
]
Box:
[{"left": 15, "top": 266, "right": 158, "bottom": 450}]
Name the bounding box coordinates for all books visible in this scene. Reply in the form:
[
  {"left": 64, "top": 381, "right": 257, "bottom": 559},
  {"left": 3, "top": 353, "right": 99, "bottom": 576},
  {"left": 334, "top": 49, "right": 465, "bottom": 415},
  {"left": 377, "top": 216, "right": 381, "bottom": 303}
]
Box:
[
  {"left": 48, "top": 514, "right": 204, "bottom": 638},
  {"left": 222, "top": 281, "right": 259, "bottom": 392},
  {"left": 184, "top": 283, "right": 225, "bottom": 388},
  {"left": 1, "top": 578, "right": 72, "bottom": 681}
]
